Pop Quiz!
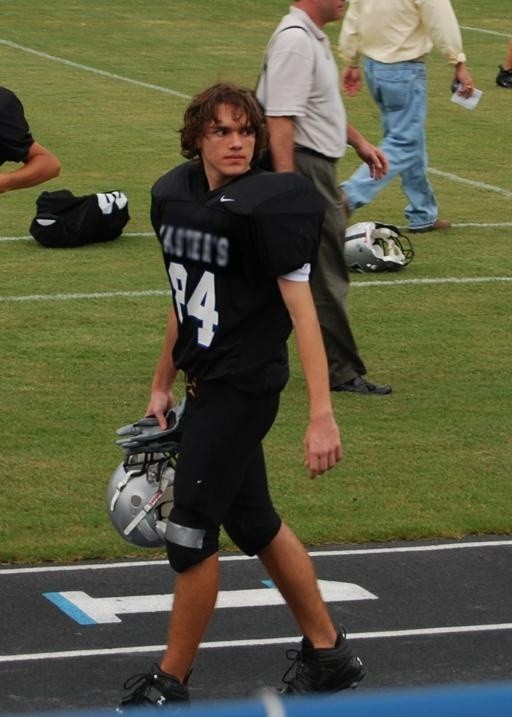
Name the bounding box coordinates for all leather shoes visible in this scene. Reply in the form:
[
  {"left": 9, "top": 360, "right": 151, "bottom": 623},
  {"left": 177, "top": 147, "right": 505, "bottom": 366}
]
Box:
[{"left": 328, "top": 374, "right": 391, "bottom": 395}]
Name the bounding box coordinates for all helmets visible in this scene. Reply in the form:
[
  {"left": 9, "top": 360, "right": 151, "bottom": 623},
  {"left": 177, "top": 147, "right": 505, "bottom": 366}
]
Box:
[
  {"left": 344, "top": 222, "right": 407, "bottom": 273},
  {"left": 105, "top": 452, "right": 177, "bottom": 547}
]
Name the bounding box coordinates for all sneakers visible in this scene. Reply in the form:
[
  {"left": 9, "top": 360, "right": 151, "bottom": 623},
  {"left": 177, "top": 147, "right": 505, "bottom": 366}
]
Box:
[
  {"left": 409, "top": 220, "right": 450, "bottom": 232},
  {"left": 116, "top": 663, "right": 193, "bottom": 713},
  {"left": 277, "top": 624, "right": 366, "bottom": 697},
  {"left": 334, "top": 187, "right": 353, "bottom": 224},
  {"left": 496, "top": 65, "right": 511, "bottom": 89}
]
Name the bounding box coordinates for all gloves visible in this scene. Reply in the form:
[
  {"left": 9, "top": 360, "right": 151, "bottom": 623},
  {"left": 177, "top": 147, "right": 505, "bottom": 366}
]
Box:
[{"left": 116, "top": 395, "right": 188, "bottom": 448}]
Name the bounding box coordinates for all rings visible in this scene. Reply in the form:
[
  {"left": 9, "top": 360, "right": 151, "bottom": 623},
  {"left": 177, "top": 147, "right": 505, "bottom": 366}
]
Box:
[{"left": 468, "top": 86, "right": 472, "bottom": 88}]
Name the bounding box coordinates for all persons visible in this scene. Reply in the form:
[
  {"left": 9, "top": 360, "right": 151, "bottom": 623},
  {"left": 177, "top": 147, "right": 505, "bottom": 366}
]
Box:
[
  {"left": 0, "top": 87, "right": 61, "bottom": 193},
  {"left": 113, "top": 83, "right": 365, "bottom": 715},
  {"left": 496, "top": 43, "right": 512, "bottom": 87},
  {"left": 337, "top": 1, "right": 474, "bottom": 232},
  {"left": 253, "top": 1, "right": 392, "bottom": 395}
]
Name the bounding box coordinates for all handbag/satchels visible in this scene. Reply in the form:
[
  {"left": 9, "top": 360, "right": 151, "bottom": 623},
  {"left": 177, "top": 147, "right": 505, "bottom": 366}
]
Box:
[{"left": 30, "top": 190, "right": 129, "bottom": 248}]
